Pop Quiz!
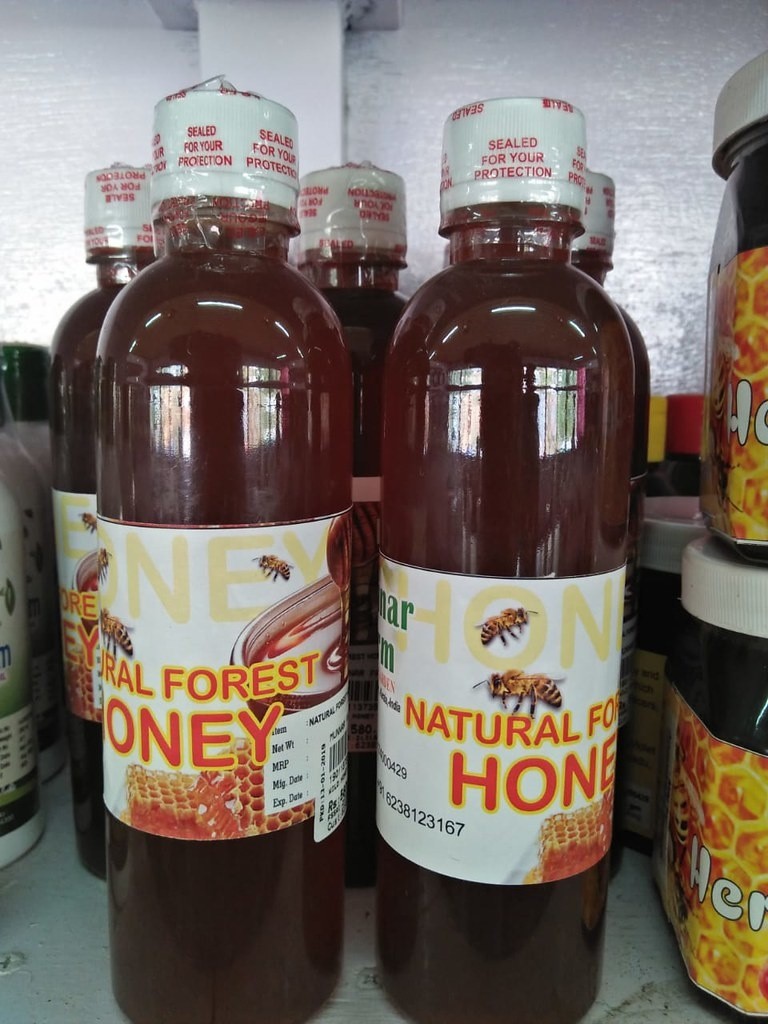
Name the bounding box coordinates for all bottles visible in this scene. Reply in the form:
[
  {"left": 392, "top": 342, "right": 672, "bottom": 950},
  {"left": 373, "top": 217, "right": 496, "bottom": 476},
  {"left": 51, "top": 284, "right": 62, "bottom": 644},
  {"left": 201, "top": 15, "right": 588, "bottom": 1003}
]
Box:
[
  {"left": 0, "top": 354, "right": 67, "bottom": 785},
  {"left": 0, "top": 343, "right": 53, "bottom": 488},
  {"left": 1, "top": 480, "right": 43, "bottom": 871},
  {"left": 570, "top": 171, "right": 650, "bottom": 878},
  {"left": 298, "top": 167, "right": 409, "bottom": 887},
  {"left": 379, "top": 100, "right": 634, "bottom": 1024},
  {"left": 49, "top": 165, "right": 155, "bottom": 880},
  {"left": 95, "top": 92, "right": 354, "bottom": 1023}
]
